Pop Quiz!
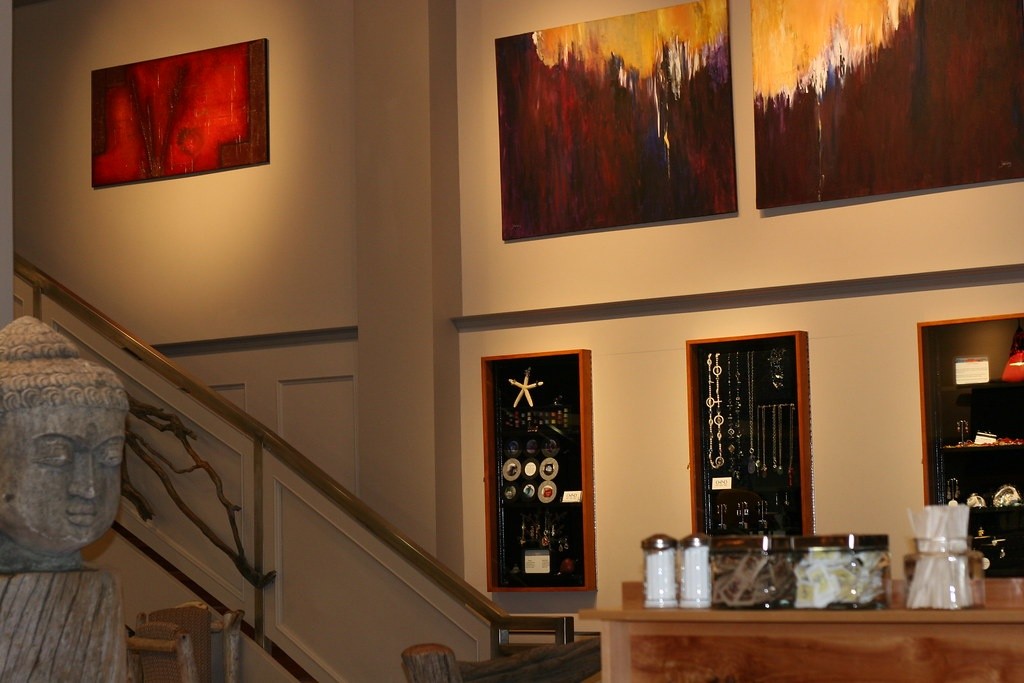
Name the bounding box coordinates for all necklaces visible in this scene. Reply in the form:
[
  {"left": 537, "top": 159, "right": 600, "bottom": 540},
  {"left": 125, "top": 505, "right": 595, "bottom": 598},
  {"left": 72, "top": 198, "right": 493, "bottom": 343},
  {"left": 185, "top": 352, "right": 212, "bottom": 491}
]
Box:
[{"left": 703, "top": 350, "right": 794, "bottom": 537}]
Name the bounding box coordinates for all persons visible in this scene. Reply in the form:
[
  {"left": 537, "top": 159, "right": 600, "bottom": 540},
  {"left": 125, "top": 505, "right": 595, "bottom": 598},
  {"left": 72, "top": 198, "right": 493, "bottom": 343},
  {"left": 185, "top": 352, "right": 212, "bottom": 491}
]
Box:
[{"left": 0, "top": 316, "right": 129, "bottom": 683}]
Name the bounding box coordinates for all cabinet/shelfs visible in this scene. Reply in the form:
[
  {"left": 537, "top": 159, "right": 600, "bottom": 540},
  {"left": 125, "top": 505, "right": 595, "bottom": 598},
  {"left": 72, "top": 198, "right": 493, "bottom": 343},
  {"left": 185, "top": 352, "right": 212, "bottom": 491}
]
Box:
[{"left": 578, "top": 602, "right": 1024, "bottom": 683}]
